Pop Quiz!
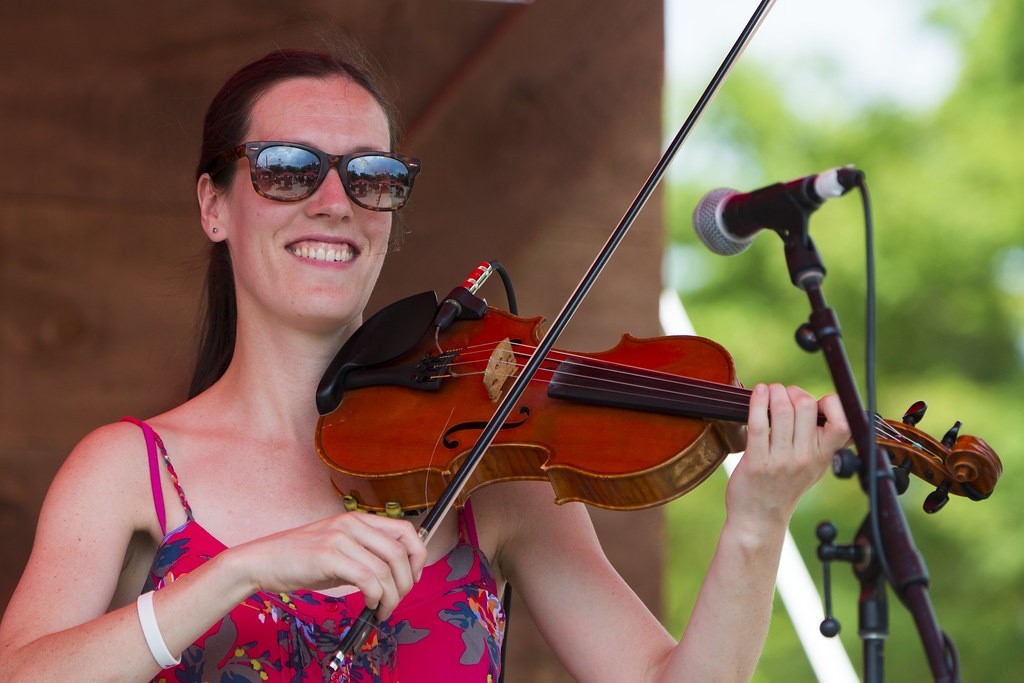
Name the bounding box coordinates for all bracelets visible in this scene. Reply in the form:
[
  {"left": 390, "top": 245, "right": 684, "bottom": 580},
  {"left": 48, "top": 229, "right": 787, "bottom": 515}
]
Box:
[{"left": 136, "top": 589, "right": 182, "bottom": 671}]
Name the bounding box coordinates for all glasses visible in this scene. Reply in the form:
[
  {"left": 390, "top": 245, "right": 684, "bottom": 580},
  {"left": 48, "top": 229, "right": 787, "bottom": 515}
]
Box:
[{"left": 237, "top": 140, "right": 421, "bottom": 214}]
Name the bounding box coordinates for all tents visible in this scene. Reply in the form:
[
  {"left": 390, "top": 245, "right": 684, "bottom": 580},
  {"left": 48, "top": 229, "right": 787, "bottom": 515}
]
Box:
[
  {"left": 257, "top": 166, "right": 317, "bottom": 178},
  {"left": 350, "top": 178, "right": 403, "bottom": 185}
]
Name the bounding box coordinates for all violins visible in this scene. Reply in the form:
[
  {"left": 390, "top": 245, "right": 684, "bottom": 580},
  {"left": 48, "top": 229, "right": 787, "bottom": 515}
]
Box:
[{"left": 314, "top": 304, "right": 1003, "bottom": 513}]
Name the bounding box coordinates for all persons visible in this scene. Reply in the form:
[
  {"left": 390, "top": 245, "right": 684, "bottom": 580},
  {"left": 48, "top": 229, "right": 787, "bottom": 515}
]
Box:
[
  {"left": 262, "top": 174, "right": 318, "bottom": 191},
  {"left": 351, "top": 182, "right": 404, "bottom": 197},
  {"left": 0, "top": 48, "right": 852, "bottom": 683}
]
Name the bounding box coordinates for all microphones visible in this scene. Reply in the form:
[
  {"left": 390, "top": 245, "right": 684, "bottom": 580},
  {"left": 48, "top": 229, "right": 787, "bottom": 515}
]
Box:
[{"left": 692, "top": 166, "right": 862, "bottom": 257}]
